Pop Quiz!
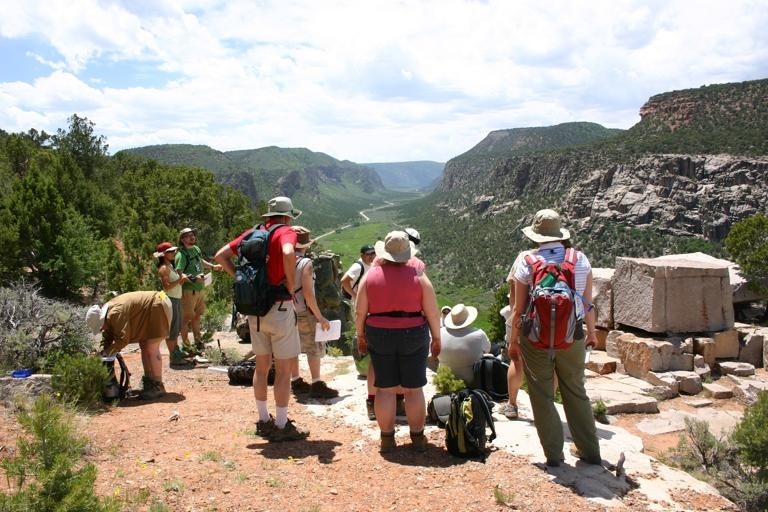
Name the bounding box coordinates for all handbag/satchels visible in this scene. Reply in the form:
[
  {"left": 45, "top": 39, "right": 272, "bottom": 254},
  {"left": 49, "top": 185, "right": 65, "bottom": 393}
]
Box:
[{"left": 227, "top": 361, "right": 276, "bottom": 386}]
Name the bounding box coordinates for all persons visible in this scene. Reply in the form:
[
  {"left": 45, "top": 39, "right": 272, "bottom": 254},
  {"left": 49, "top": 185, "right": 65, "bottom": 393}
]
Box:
[
  {"left": 288, "top": 222, "right": 334, "bottom": 407},
  {"left": 435, "top": 301, "right": 491, "bottom": 393},
  {"left": 506, "top": 207, "right": 607, "bottom": 469},
  {"left": 174, "top": 224, "right": 224, "bottom": 358},
  {"left": 338, "top": 241, "right": 377, "bottom": 384},
  {"left": 213, "top": 192, "right": 311, "bottom": 443},
  {"left": 351, "top": 228, "right": 440, "bottom": 454},
  {"left": 497, "top": 248, "right": 560, "bottom": 419},
  {"left": 360, "top": 226, "right": 424, "bottom": 421},
  {"left": 83, "top": 286, "right": 173, "bottom": 407},
  {"left": 152, "top": 240, "right": 191, "bottom": 371}
]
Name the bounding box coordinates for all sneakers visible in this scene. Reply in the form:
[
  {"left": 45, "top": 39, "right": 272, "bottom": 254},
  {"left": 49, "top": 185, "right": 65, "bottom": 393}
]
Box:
[
  {"left": 410, "top": 434, "right": 428, "bottom": 452},
  {"left": 496, "top": 401, "right": 519, "bottom": 419},
  {"left": 169, "top": 341, "right": 205, "bottom": 369},
  {"left": 138, "top": 380, "right": 165, "bottom": 400},
  {"left": 546, "top": 451, "right": 565, "bottom": 467},
  {"left": 309, "top": 381, "right": 339, "bottom": 398},
  {"left": 569, "top": 443, "right": 602, "bottom": 464},
  {"left": 255, "top": 416, "right": 276, "bottom": 436},
  {"left": 291, "top": 377, "right": 311, "bottom": 395},
  {"left": 380, "top": 431, "right": 398, "bottom": 452},
  {"left": 269, "top": 421, "right": 307, "bottom": 442},
  {"left": 366, "top": 398, "right": 407, "bottom": 420}
]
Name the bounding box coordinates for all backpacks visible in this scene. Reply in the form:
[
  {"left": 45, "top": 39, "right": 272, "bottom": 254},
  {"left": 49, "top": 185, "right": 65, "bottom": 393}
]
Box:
[
  {"left": 312, "top": 250, "right": 343, "bottom": 321},
  {"left": 428, "top": 394, "right": 452, "bottom": 429},
  {"left": 472, "top": 353, "right": 509, "bottom": 404},
  {"left": 232, "top": 224, "right": 272, "bottom": 317},
  {"left": 446, "top": 387, "right": 495, "bottom": 461},
  {"left": 523, "top": 248, "right": 581, "bottom": 352}
]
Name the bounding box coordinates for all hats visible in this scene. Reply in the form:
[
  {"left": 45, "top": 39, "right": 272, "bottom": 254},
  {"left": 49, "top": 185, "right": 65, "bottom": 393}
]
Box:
[
  {"left": 375, "top": 230, "right": 416, "bottom": 263},
  {"left": 85, "top": 303, "right": 109, "bottom": 335},
  {"left": 360, "top": 244, "right": 376, "bottom": 254},
  {"left": 179, "top": 227, "right": 197, "bottom": 237},
  {"left": 521, "top": 208, "right": 571, "bottom": 243},
  {"left": 405, "top": 228, "right": 421, "bottom": 245},
  {"left": 261, "top": 196, "right": 302, "bottom": 220},
  {"left": 444, "top": 303, "right": 479, "bottom": 330},
  {"left": 291, "top": 225, "right": 315, "bottom": 250},
  {"left": 156, "top": 243, "right": 178, "bottom": 256}
]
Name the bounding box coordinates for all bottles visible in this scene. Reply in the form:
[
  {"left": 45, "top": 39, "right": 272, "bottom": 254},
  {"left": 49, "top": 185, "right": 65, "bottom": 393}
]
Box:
[
  {"left": 105, "top": 381, "right": 117, "bottom": 398},
  {"left": 5, "top": 369, "right": 30, "bottom": 378},
  {"left": 235, "top": 271, "right": 244, "bottom": 282}
]
